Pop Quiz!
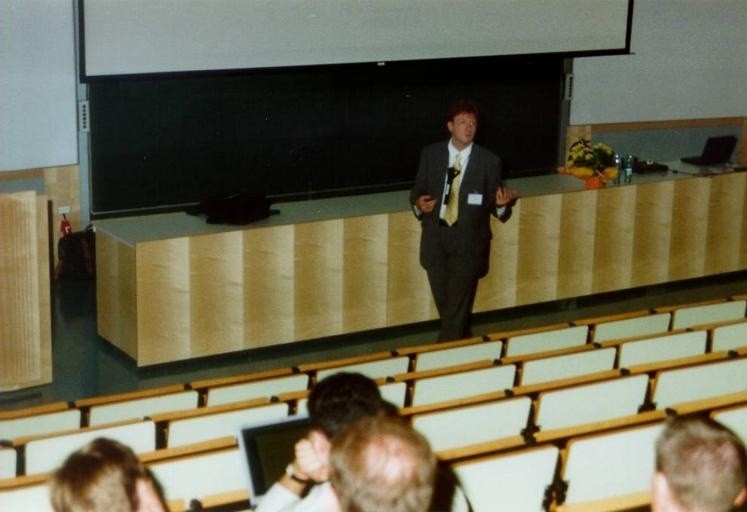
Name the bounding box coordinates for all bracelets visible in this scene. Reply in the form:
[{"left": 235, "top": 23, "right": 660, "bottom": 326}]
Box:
[{"left": 284, "top": 464, "right": 310, "bottom": 486}]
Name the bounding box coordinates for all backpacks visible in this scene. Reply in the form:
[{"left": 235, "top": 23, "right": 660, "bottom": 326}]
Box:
[{"left": 186, "top": 158, "right": 280, "bottom": 226}]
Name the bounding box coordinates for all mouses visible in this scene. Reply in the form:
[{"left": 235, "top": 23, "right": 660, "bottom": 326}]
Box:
[{"left": 646, "top": 159, "right": 654, "bottom": 165}]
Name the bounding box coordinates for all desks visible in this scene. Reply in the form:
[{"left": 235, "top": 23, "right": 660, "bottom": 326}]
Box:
[{"left": 91, "top": 162, "right": 746, "bottom": 366}]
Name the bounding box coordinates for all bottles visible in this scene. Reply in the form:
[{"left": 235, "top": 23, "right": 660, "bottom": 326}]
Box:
[{"left": 611, "top": 152, "right": 634, "bottom": 176}]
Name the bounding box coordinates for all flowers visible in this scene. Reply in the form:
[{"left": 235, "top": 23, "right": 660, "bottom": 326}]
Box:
[{"left": 557, "top": 138, "right": 618, "bottom": 189}]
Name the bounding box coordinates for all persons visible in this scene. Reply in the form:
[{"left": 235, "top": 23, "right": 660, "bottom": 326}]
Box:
[
  {"left": 49, "top": 437, "right": 170, "bottom": 512},
  {"left": 648, "top": 410, "right": 746, "bottom": 512},
  {"left": 326, "top": 410, "right": 437, "bottom": 512},
  {"left": 408, "top": 98, "right": 520, "bottom": 346},
  {"left": 251, "top": 369, "right": 386, "bottom": 512}
]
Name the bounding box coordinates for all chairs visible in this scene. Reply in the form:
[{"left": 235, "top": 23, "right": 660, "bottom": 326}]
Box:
[{"left": 0, "top": 294, "right": 747, "bottom": 512}]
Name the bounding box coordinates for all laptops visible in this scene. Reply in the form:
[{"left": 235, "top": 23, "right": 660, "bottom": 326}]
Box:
[
  {"left": 680, "top": 135, "right": 737, "bottom": 165},
  {"left": 236, "top": 415, "right": 315, "bottom": 510},
  {"left": 630, "top": 160, "right": 668, "bottom": 174}
]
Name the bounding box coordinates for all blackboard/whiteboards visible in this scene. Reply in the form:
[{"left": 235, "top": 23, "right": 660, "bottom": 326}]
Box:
[{"left": 89, "top": 58, "right": 573, "bottom": 220}]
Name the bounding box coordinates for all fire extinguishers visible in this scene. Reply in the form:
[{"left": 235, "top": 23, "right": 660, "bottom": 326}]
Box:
[{"left": 61, "top": 213, "right": 72, "bottom": 236}]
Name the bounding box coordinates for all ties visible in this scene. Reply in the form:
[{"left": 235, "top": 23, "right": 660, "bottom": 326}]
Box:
[{"left": 443, "top": 153, "right": 463, "bottom": 227}]
